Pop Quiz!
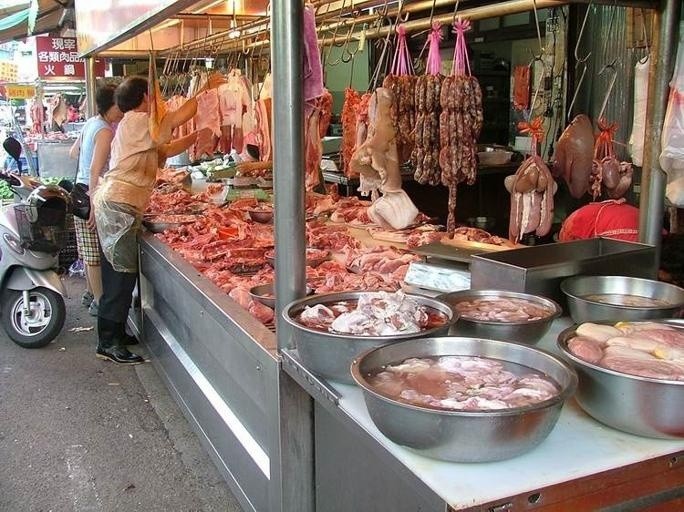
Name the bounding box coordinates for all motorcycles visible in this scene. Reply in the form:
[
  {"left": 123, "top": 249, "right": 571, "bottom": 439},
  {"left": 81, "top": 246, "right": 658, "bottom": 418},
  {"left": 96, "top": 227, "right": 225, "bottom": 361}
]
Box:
[{"left": 0, "top": 136, "right": 90, "bottom": 349}]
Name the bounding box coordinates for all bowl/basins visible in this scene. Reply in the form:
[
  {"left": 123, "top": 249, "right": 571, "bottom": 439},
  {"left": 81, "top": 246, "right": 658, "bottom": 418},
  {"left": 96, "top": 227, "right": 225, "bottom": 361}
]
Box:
[
  {"left": 435, "top": 288, "right": 562, "bottom": 347},
  {"left": 142, "top": 217, "right": 194, "bottom": 233},
  {"left": 281, "top": 289, "right": 461, "bottom": 387},
  {"left": 264, "top": 249, "right": 330, "bottom": 270},
  {"left": 555, "top": 316, "right": 684, "bottom": 441},
  {"left": 479, "top": 152, "right": 513, "bottom": 166},
  {"left": 248, "top": 280, "right": 311, "bottom": 310},
  {"left": 559, "top": 274, "right": 683, "bottom": 324},
  {"left": 349, "top": 334, "right": 578, "bottom": 464},
  {"left": 248, "top": 210, "right": 272, "bottom": 223}
]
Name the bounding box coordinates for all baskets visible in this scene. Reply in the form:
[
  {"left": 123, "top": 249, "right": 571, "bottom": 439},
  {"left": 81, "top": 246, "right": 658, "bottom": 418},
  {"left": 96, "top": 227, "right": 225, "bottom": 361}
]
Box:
[{"left": 14, "top": 204, "right": 67, "bottom": 252}]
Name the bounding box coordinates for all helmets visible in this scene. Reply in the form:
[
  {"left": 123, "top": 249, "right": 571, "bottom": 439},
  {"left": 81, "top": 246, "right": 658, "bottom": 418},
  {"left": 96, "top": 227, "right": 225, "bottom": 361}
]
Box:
[{"left": 25, "top": 185, "right": 73, "bottom": 224}]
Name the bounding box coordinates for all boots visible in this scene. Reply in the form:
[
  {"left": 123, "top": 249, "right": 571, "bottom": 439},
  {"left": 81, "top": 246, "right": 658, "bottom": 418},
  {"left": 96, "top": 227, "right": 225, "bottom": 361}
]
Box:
[{"left": 97, "top": 316, "right": 144, "bottom": 365}]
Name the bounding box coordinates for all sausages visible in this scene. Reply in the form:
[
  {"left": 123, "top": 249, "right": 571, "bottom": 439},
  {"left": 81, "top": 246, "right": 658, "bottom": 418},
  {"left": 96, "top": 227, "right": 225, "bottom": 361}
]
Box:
[{"left": 381, "top": 72, "right": 486, "bottom": 241}]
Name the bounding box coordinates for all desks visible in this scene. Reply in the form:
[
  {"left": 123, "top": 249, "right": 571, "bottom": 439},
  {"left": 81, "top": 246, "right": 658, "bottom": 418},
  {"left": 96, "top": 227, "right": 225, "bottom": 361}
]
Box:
[{"left": 322, "top": 161, "right": 519, "bottom": 197}]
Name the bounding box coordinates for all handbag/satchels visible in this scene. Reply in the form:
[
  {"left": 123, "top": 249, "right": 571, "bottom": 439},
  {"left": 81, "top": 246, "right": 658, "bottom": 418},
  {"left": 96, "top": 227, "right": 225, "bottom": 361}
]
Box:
[{"left": 69, "top": 186, "right": 92, "bottom": 221}]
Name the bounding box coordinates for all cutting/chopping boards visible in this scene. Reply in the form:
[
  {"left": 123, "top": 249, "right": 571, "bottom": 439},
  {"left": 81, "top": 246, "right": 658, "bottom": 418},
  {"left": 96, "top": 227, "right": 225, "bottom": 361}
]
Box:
[{"left": 413, "top": 239, "right": 513, "bottom": 265}]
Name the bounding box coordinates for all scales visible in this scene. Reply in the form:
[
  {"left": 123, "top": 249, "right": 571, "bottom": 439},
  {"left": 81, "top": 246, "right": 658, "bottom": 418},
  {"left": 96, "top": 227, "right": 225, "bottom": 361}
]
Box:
[
  {"left": 321, "top": 137, "right": 345, "bottom": 173},
  {"left": 403, "top": 240, "right": 514, "bottom": 292},
  {"left": 225, "top": 177, "right": 269, "bottom": 201}
]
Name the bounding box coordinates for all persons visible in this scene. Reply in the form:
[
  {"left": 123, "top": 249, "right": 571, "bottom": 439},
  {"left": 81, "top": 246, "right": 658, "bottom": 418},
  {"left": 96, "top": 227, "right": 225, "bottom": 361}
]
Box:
[
  {"left": 67, "top": 80, "right": 122, "bottom": 318},
  {"left": 92, "top": 73, "right": 230, "bottom": 366}
]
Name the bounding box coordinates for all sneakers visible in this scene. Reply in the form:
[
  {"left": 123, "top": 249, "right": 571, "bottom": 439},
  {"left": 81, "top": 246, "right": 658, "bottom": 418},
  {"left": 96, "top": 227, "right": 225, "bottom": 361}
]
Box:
[{"left": 80, "top": 289, "right": 98, "bottom": 316}]
